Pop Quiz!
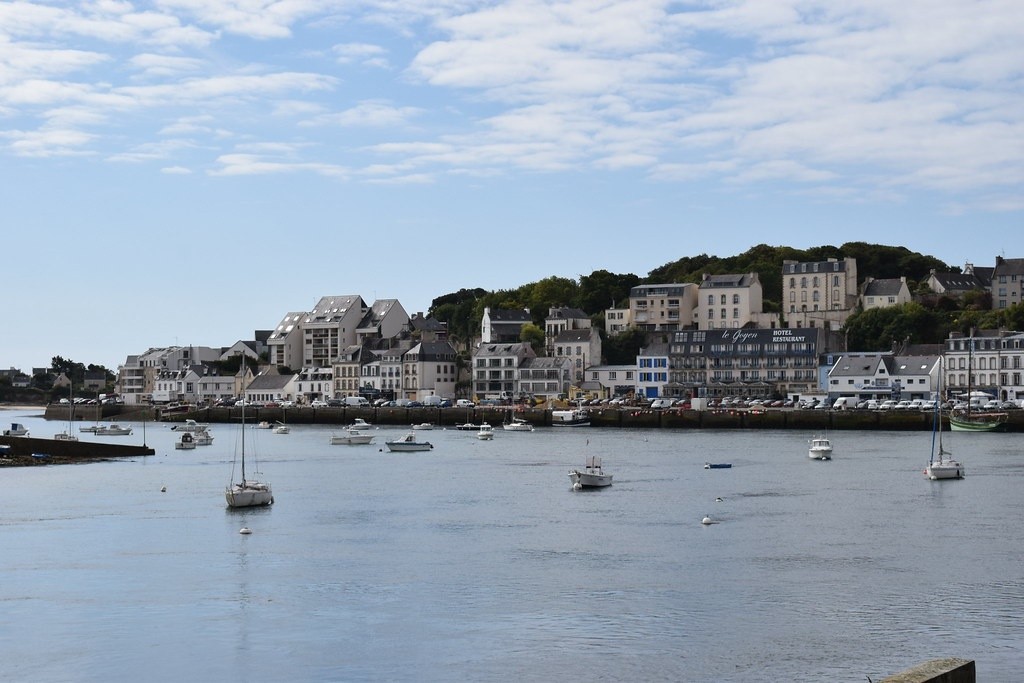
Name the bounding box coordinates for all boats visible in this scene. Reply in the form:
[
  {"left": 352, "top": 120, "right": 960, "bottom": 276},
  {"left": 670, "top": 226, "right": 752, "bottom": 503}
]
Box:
[
  {"left": 3, "top": 422, "right": 29, "bottom": 436},
  {"left": 704, "top": 462, "right": 733, "bottom": 469},
  {"left": 258, "top": 422, "right": 274, "bottom": 429},
  {"left": 456, "top": 422, "right": 482, "bottom": 430},
  {"left": 330, "top": 429, "right": 376, "bottom": 444},
  {"left": 550, "top": 409, "right": 592, "bottom": 427},
  {"left": 808, "top": 435, "right": 834, "bottom": 460},
  {"left": 192, "top": 429, "right": 215, "bottom": 446},
  {"left": 502, "top": 422, "right": 535, "bottom": 432},
  {"left": 343, "top": 418, "right": 372, "bottom": 431},
  {"left": 272, "top": 425, "right": 291, "bottom": 434},
  {"left": 385, "top": 433, "right": 434, "bottom": 451},
  {"left": 176, "top": 418, "right": 210, "bottom": 433},
  {"left": 95, "top": 424, "right": 133, "bottom": 436},
  {"left": 575, "top": 455, "right": 614, "bottom": 490},
  {"left": 175, "top": 431, "right": 197, "bottom": 449},
  {"left": 413, "top": 422, "right": 434, "bottom": 430},
  {"left": 477, "top": 424, "right": 495, "bottom": 441}
]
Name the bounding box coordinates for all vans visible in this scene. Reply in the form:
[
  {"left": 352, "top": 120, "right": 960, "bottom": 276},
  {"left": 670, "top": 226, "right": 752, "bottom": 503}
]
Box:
[{"left": 456, "top": 399, "right": 475, "bottom": 407}]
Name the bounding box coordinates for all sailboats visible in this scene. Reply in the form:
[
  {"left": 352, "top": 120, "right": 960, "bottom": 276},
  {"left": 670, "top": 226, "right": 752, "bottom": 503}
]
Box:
[
  {"left": 79, "top": 393, "right": 106, "bottom": 432},
  {"left": 225, "top": 349, "right": 274, "bottom": 507},
  {"left": 948, "top": 337, "right": 1009, "bottom": 431},
  {"left": 924, "top": 355, "right": 967, "bottom": 481},
  {"left": 53, "top": 377, "right": 80, "bottom": 441}
]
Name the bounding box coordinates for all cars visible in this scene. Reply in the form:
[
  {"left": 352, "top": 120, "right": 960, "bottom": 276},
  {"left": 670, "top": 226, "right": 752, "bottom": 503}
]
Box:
[
  {"left": 568, "top": 398, "right": 692, "bottom": 410},
  {"left": 214, "top": 396, "right": 453, "bottom": 408},
  {"left": 705, "top": 395, "right": 1020, "bottom": 410},
  {"left": 59, "top": 392, "right": 125, "bottom": 404}
]
